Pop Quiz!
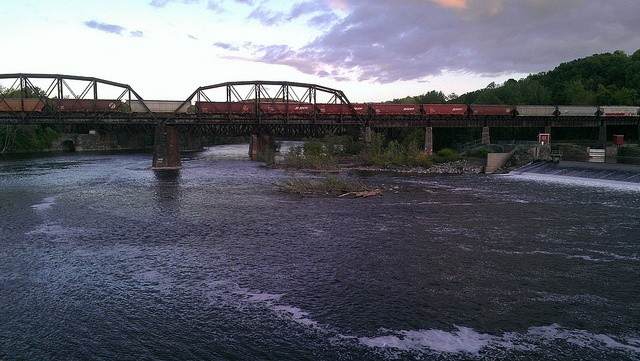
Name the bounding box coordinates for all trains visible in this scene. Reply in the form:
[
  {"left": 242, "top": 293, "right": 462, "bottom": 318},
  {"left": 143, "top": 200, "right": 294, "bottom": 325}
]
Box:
[{"left": 0, "top": 96, "right": 640, "bottom": 119}]
